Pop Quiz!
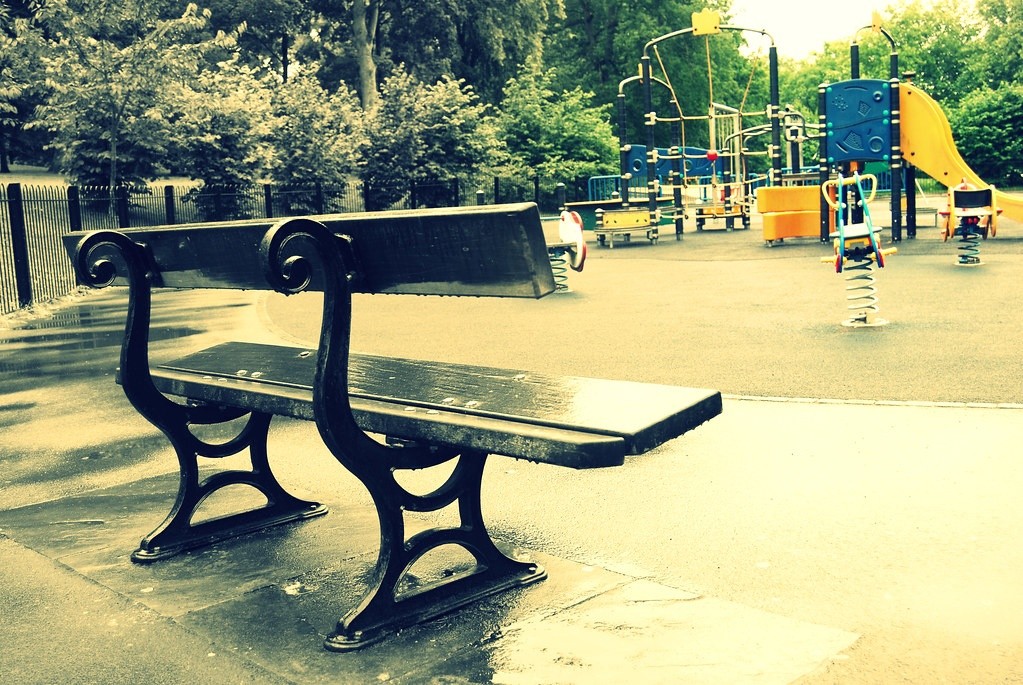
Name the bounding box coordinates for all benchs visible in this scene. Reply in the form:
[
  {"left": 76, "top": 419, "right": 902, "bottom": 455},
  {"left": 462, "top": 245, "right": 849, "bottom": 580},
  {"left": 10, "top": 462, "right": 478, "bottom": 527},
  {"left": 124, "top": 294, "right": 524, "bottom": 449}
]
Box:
[
  {"left": 61, "top": 204, "right": 723, "bottom": 654},
  {"left": 901, "top": 207, "right": 938, "bottom": 227}
]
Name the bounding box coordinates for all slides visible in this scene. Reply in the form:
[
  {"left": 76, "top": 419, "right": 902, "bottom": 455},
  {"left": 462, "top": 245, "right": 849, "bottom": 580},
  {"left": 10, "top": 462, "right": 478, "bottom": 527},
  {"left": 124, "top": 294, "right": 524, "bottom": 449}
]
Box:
[{"left": 898, "top": 83, "right": 1023, "bottom": 223}]
